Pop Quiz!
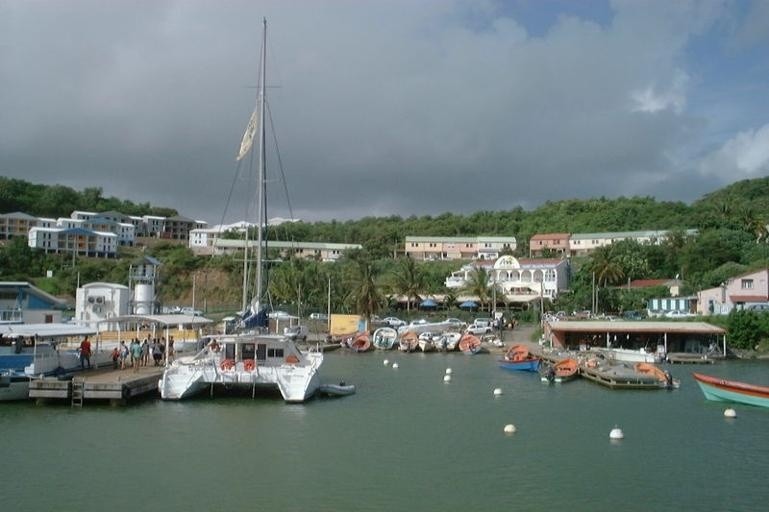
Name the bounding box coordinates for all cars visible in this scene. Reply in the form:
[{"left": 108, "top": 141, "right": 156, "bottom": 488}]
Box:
[
  {"left": 740, "top": 302, "right": 769, "bottom": 310},
  {"left": 310, "top": 311, "right": 496, "bottom": 334},
  {"left": 545, "top": 308, "right": 699, "bottom": 320},
  {"left": 181, "top": 306, "right": 203, "bottom": 317}
]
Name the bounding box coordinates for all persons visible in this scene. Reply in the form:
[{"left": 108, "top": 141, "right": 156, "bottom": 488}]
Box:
[
  {"left": 109, "top": 333, "right": 175, "bottom": 370},
  {"left": 76, "top": 335, "right": 92, "bottom": 369}
]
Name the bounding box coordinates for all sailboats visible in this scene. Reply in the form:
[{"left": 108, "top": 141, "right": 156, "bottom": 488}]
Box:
[{"left": 154, "top": 16, "right": 327, "bottom": 407}]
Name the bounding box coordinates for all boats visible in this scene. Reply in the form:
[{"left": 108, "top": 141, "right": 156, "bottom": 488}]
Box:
[
  {"left": 496, "top": 343, "right": 541, "bottom": 372},
  {"left": 318, "top": 383, "right": 356, "bottom": 399},
  {"left": 0, "top": 280, "right": 124, "bottom": 402},
  {"left": 338, "top": 327, "right": 485, "bottom": 355},
  {"left": 695, "top": 370, "right": 769, "bottom": 412},
  {"left": 541, "top": 356, "right": 578, "bottom": 383}
]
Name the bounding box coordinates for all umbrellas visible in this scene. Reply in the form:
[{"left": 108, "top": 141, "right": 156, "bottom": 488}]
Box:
[
  {"left": 459, "top": 301, "right": 478, "bottom": 312},
  {"left": 420, "top": 300, "right": 437, "bottom": 306}
]
